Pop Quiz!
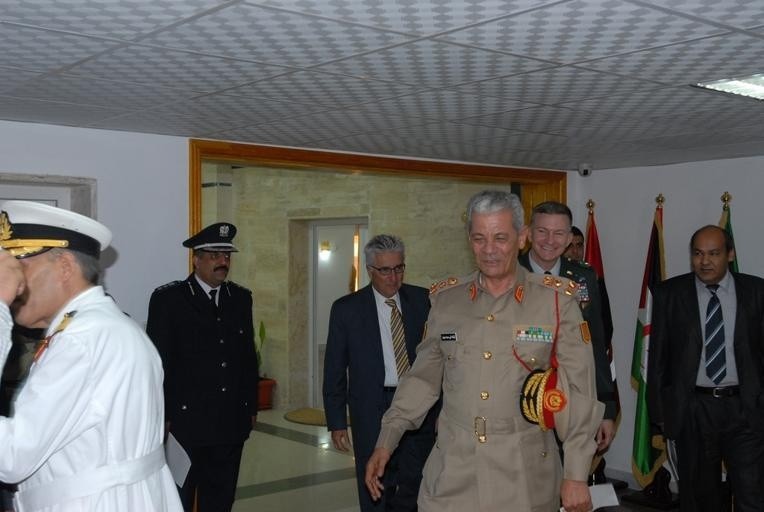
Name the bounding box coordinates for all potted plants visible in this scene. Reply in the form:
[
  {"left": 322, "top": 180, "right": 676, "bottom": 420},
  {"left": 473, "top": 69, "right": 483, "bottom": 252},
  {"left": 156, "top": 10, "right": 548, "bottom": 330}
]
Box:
[{"left": 248, "top": 318, "right": 276, "bottom": 412}]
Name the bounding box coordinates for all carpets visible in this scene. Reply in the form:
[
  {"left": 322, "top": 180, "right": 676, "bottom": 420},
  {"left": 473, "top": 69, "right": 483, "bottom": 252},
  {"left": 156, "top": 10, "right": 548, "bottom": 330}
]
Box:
[{"left": 283, "top": 406, "right": 351, "bottom": 427}]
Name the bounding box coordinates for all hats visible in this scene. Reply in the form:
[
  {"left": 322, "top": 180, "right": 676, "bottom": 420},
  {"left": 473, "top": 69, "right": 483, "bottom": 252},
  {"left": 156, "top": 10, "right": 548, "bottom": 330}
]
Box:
[
  {"left": 520, "top": 364, "right": 566, "bottom": 429},
  {"left": 0, "top": 198, "right": 113, "bottom": 258},
  {"left": 183, "top": 223, "right": 241, "bottom": 253}
]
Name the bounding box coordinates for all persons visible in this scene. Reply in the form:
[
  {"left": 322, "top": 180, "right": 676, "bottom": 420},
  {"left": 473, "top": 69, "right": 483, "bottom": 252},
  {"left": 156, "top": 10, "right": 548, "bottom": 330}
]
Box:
[
  {"left": 513, "top": 198, "right": 617, "bottom": 484},
  {"left": 0, "top": 196, "right": 186, "bottom": 512},
  {"left": 0, "top": 247, "right": 29, "bottom": 383},
  {"left": 359, "top": 189, "right": 607, "bottom": 511},
  {"left": 143, "top": 219, "right": 263, "bottom": 512},
  {"left": 643, "top": 222, "right": 764, "bottom": 511},
  {"left": 561, "top": 223, "right": 614, "bottom": 350},
  {"left": 319, "top": 231, "right": 446, "bottom": 512}
]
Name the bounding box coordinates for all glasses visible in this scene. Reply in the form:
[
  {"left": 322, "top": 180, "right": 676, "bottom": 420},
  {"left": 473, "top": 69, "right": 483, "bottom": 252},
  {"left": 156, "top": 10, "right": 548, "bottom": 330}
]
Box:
[{"left": 367, "top": 263, "right": 405, "bottom": 276}]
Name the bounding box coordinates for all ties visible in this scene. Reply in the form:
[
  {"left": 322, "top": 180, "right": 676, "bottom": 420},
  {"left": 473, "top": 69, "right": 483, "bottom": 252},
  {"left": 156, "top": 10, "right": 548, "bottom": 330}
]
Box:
[
  {"left": 208, "top": 289, "right": 217, "bottom": 304},
  {"left": 385, "top": 297, "right": 411, "bottom": 380},
  {"left": 704, "top": 283, "right": 727, "bottom": 384}
]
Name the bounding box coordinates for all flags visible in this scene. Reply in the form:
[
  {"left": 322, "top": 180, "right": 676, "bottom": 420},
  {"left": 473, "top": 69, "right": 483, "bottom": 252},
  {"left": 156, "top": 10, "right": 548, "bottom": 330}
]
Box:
[
  {"left": 628, "top": 191, "right": 670, "bottom": 489},
  {"left": 712, "top": 191, "right": 739, "bottom": 277},
  {"left": 580, "top": 198, "right": 622, "bottom": 477}
]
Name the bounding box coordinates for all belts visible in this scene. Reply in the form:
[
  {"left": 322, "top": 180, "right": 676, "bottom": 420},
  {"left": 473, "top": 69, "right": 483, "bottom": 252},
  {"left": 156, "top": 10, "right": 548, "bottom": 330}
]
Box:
[{"left": 694, "top": 385, "right": 738, "bottom": 397}]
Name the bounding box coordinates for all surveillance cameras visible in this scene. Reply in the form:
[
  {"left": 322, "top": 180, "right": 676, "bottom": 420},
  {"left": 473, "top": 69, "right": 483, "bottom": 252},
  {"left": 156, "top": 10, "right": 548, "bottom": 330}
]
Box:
[{"left": 578, "top": 163, "right": 592, "bottom": 177}]
